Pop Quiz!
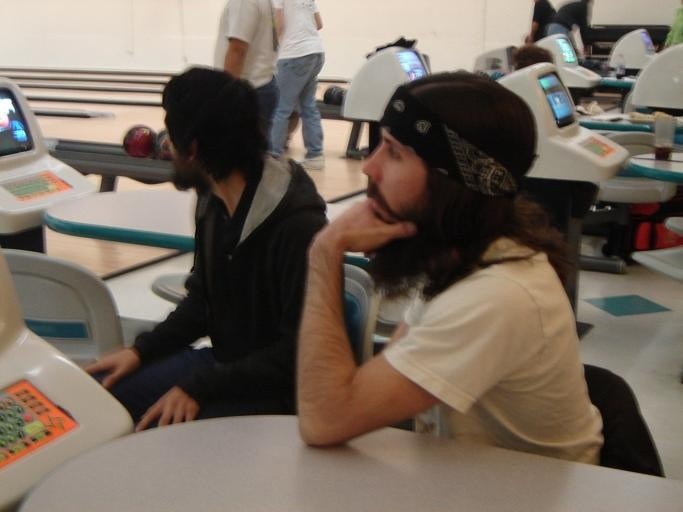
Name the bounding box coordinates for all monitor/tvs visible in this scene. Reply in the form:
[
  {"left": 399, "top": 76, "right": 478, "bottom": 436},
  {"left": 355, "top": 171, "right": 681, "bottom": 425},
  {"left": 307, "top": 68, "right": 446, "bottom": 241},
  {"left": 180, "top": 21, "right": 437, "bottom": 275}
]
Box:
[
  {"left": 537, "top": 69, "right": 580, "bottom": 131},
  {"left": 640, "top": 31, "right": 655, "bottom": 55},
  {"left": 395, "top": 49, "right": 428, "bottom": 84},
  {"left": 555, "top": 36, "right": 580, "bottom": 63},
  {"left": 0, "top": 82, "right": 36, "bottom": 158}
]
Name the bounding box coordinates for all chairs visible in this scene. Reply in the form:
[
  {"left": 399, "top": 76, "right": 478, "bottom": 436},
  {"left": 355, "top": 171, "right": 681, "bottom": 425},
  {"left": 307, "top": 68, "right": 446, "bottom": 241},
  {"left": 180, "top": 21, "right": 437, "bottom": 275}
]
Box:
[
  {"left": 17, "top": 244, "right": 164, "bottom": 387},
  {"left": 152, "top": 272, "right": 194, "bottom": 304},
  {"left": 335, "top": 250, "right": 417, "bottom": 368}
]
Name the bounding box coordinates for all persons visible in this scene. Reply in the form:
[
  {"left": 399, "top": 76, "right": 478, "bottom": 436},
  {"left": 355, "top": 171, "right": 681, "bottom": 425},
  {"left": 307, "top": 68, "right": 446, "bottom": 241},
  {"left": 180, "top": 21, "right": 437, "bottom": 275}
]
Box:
[
  {"left": 545, "top": 1, "right": 593, "bottom": 64},
  {"left": 269, "top": 0, "right": 328, "bottom": 172},
  {"left": 524, "top": 1, "right": 557, "bottom": 46},
  {"left": 83, "top": 63, "right": 330, "bottom": 431},
  {"left": 211, "top": 0, "right": 281, "bottom": 154},
  {"left": 511, "top": 45, "right": 555, "bottom": 74},
  {"left": 293, "top": 68, "right": 606, "bottom": 465}
]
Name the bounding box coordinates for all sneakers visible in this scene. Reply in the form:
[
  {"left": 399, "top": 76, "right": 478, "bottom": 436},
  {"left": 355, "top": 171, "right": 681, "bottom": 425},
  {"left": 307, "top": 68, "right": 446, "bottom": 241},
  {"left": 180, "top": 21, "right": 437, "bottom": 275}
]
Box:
[{"left": 298, "top": 155, "right": 326, "bottom": 170}]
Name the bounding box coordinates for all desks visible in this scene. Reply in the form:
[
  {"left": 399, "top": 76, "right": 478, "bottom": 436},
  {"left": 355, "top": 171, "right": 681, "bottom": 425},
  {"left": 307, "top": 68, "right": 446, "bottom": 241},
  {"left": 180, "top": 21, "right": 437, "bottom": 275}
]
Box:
[
  {"left": 17, "top": 409, "right": 683, "bottom": 510},
  {"left": 43, "top": 179, "right": 372, "bottom": 271}
]
